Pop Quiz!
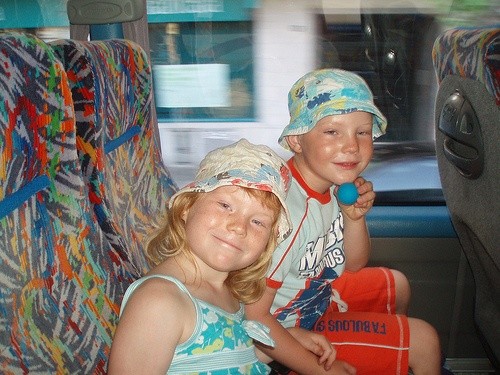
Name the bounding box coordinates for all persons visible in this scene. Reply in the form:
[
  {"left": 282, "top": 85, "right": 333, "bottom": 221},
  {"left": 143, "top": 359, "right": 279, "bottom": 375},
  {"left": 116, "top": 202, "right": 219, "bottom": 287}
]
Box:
[
  {"left": 243, "top": 69, "right": 443, "bottom": 375},
  {"left": 108, "top": 141, "right": 337, "bottom": 375}
]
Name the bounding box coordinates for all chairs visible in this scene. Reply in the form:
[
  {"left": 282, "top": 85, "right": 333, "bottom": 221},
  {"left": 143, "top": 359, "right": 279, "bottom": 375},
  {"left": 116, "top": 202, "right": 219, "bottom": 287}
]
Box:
[
  {"left": 432, "top": 24, "right": 500, "bottom": 361},
  {"left": 0, "top": 28, "right": 136, "bottom": 375},
  {"left": 46, "top": 38, "right": 179, "bottom": 277}
]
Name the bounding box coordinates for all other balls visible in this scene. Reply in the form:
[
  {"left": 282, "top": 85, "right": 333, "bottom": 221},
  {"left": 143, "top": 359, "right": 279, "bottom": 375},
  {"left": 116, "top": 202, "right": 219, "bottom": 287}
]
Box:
[{"left": 337, "top": 183, "right": 359, "bottom": 206}]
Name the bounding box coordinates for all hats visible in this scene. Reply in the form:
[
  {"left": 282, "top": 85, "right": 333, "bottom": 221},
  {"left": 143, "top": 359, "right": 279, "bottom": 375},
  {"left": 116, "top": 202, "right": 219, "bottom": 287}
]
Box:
[
  {"left": 278, "top": 69, "right": 388, "bottom": 153},
  {"left": 168, "top": 138, "right": 293, "bottom": 244}
]
